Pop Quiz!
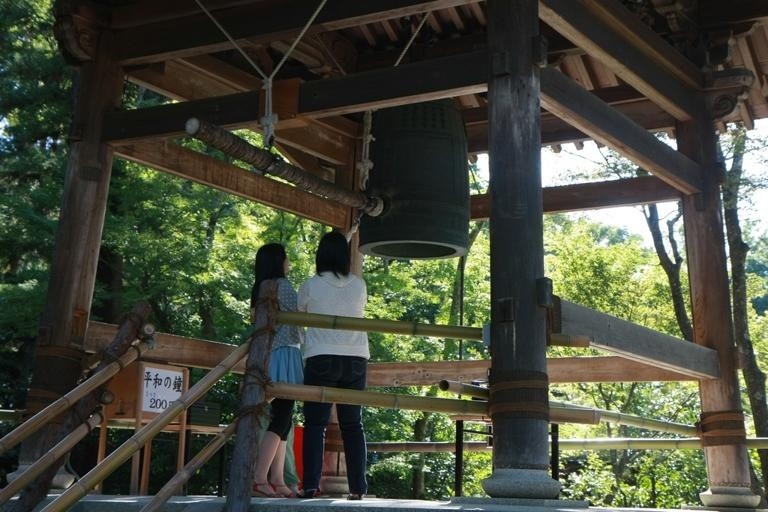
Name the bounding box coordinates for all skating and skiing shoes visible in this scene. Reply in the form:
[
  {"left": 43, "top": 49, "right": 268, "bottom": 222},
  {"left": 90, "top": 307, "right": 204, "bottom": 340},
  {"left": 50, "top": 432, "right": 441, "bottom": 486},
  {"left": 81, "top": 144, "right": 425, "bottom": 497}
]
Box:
[
  {"left": 347, "top": 489, "right": 363, "bottom": 500},
  {"left": 300, "top": 488, "right": 317, "bottom": 498}
]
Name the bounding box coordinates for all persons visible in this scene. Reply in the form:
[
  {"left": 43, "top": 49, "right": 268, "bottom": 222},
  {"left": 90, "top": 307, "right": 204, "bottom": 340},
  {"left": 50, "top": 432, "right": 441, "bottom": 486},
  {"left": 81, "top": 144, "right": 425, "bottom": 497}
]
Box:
[
  {"left": 249, "top": 242, "right": 300, "bottom": 498},
  {"left": 298, "top": 231, "right": 372, "bottom": 500},
  {"left": 258, "top": 401, "right": 300, "bottom": 494}
]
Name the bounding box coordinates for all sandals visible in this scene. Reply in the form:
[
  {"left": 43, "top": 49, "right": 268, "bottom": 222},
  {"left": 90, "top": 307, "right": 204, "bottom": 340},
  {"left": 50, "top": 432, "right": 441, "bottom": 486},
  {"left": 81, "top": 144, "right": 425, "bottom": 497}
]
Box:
[{"left": 251, "top": 482, "right": 302, "bottom": 498}]
